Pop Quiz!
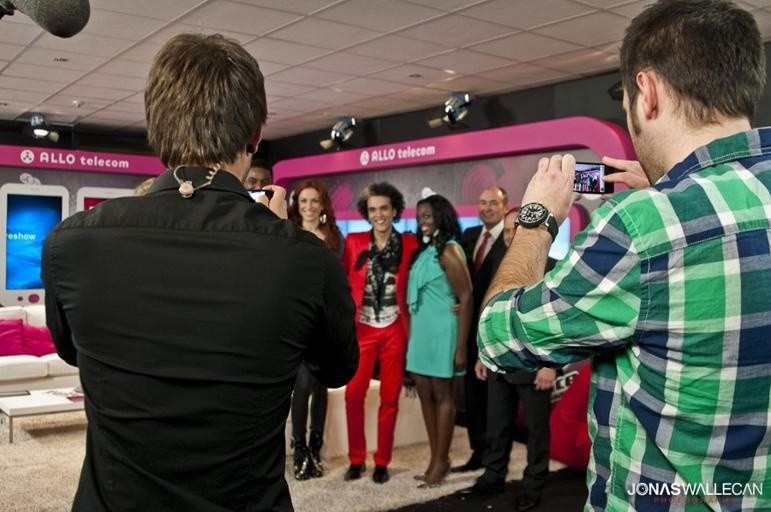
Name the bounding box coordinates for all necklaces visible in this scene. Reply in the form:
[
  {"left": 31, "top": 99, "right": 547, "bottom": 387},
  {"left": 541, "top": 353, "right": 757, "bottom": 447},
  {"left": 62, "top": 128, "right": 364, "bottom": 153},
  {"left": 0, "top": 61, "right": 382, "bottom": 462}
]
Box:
[{"left": 173, "top": 162, "right": 222, "bottom": 198}]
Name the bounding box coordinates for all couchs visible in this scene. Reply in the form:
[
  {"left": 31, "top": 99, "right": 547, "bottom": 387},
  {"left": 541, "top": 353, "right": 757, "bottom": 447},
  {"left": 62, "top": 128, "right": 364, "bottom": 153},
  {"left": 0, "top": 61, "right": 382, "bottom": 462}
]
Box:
[{"left": 0, "top": 305, "right": 90, "bottom": 379}]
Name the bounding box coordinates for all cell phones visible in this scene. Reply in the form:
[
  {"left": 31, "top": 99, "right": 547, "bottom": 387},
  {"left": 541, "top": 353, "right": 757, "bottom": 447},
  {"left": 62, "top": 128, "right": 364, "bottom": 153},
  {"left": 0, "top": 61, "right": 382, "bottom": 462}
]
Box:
[
  {"left": 563, "top": 159, "right": 616, "bottom": 196},
  {"left": 245, "top": 187, "right": 274, "bottom": 207}
]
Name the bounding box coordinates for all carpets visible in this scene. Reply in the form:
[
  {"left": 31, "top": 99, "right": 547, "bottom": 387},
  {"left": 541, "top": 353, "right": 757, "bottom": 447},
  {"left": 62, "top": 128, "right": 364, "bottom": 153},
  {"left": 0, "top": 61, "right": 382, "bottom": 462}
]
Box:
[{"left": 0, "top": 379, "right": 570, "bottom": 512}]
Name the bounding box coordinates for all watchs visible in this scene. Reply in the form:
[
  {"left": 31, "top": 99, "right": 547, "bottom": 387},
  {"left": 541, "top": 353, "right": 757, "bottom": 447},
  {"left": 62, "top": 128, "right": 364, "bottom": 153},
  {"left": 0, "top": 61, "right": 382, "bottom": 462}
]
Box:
[{"left": 514, "top": 202, "right": 558, "bottom": 242}]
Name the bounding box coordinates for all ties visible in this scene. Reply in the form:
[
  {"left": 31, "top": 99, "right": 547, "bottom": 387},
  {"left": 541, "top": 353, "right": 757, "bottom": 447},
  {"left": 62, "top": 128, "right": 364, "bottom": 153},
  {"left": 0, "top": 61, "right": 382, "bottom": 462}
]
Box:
[{"left": 472, "top": 230, "right": 493, "bottom": 273}]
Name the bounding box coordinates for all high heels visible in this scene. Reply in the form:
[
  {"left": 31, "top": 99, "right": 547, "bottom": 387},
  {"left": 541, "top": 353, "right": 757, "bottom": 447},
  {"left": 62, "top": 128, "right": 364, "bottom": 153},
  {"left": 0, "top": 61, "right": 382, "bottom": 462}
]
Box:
[
  {"left": 416, "top": 458, "right": 451, "bottom": 490},
  {"left": 412, "top": 471, "right": 434, "bottom": 482}
]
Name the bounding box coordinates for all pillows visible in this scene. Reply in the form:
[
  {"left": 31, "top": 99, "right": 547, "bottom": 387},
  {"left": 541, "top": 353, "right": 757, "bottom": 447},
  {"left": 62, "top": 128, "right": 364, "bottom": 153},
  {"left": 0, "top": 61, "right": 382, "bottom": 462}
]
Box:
[{"left": 0, "top": 318, "right": 58, "bottom": 357}]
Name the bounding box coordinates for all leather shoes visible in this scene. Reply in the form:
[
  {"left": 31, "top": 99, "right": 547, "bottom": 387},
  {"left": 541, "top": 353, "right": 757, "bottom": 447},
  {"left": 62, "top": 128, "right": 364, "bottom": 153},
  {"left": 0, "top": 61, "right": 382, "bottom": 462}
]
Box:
[
  {"left": 372, "top": 463, "right": 392, "bottom": 486},
  {"left": 292, "top": 446, "right": 312, "bottom": 480},
  {"left": 449, "top": 454, "right": 487, "bottom": 474},
  {"left": 306, "top": 446, "right": 324, "bottom": 481},
  {"left": 342, "top": 460, "right": 367, "bottom": 481},
  {"left": 514, "top": 485, "right": 544, "bottom": 512},
  {"left": 454, "top": 480, "right": 508, "bottom": 500}
]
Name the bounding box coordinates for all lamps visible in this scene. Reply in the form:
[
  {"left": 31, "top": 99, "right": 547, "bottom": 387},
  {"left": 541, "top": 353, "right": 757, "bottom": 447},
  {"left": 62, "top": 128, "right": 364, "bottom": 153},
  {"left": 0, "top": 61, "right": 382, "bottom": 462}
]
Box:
[
  {"left": 30, "top": 111, "right": 52, "bottom": 139},
  {"left": 329, "top": 115, "right": 357, "bottom": 144},
  {"left": 442, "top": 87, "right": 470, "bottom": 125}
]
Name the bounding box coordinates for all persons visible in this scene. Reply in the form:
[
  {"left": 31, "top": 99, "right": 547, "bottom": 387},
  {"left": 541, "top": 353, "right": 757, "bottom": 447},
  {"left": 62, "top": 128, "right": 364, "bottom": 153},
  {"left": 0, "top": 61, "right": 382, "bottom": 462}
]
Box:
[
  {"left": 135, "top": 176, "right": 155, "bottom": 195},
  {"left": 451, "top": 186, "right": 510, "bottom": 471},
  {"left": 475, "top": 0, "right": 770, "bottom": 512},
  {"left": 462, "top": 206, "right": 559, "bottom": 512},
  {"left": 287, "top": 180, "right": 346, "bottom": 481},
  {"left": 341, "top": 180, "right": 420, "bottom": 483},
  {"left": 40, "top": 34, "right": 360, "bottom": 511},
  {"left": 242, "top": 159, "right": 271, "bottom": 191},
  {"left": 573, "top": 171, "right": 600, "bottom": 191},
  {"left": 405, "top": 195, "right": 474, "bottom": 487}
]
